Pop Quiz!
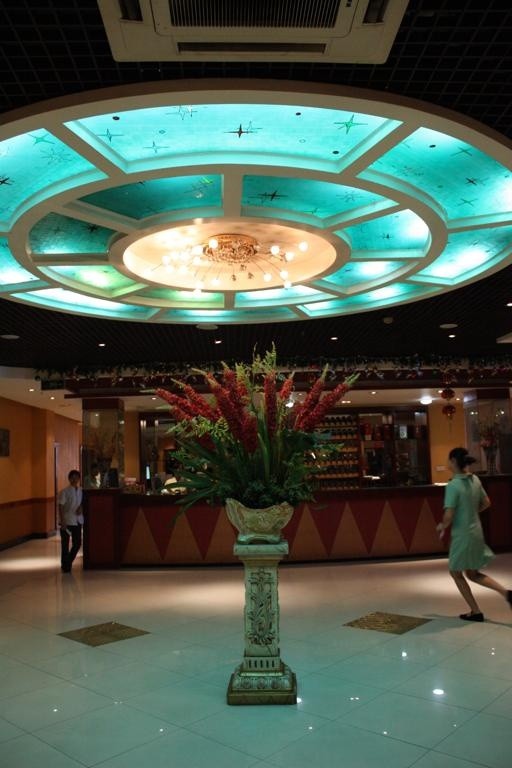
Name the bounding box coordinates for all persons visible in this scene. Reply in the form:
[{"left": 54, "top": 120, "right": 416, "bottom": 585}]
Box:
[
  {"left": 369, "top": 447, "right": 399, "bottom": 485},
  {"left": 437, "top": 448, "right": 512, "bottom": 622},
  {"left": 84, "top": 463, "right": 99, "bottom": 489},
  {"left": 162, "top": 462, "right": 195, "bottom": 496},
  {"left": 58, "top": 469, "right": 84, "bottom": 572}
]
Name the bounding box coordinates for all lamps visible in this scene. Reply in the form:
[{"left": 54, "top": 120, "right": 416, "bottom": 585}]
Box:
[
  {"left": 159, "top": 233, "right": 308, "bottom": 296},
  {"left": 420, "top": 399, "right": 432, "bottom": 405}
]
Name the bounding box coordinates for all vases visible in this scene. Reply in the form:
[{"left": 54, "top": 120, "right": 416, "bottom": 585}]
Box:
[
  {"left": 482, "top": 446, "right": 497, "bottom": 475},
  {"left": 224, "top": 496, "right": 294, "bottom": 544}
]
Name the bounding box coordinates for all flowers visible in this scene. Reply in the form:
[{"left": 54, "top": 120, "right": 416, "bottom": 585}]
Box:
[
  {"left": 157, "top": 343, "right": 361, "bottom": 522},
  {"left": 471, "top": 407, "right": 502, "bottom": 449}
]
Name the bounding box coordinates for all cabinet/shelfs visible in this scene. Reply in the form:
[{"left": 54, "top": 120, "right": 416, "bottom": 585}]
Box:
[{"left": 311, "top": 406, "right": 427, "bottom": 489}]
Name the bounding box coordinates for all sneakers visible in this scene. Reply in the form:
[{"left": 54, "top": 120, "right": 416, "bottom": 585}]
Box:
[
  {"left": 507, "top": 589, "right": 512, "bottom": 609},
  {"left": 460, "top": 612, "right": 483, "bottom": 621}
]
